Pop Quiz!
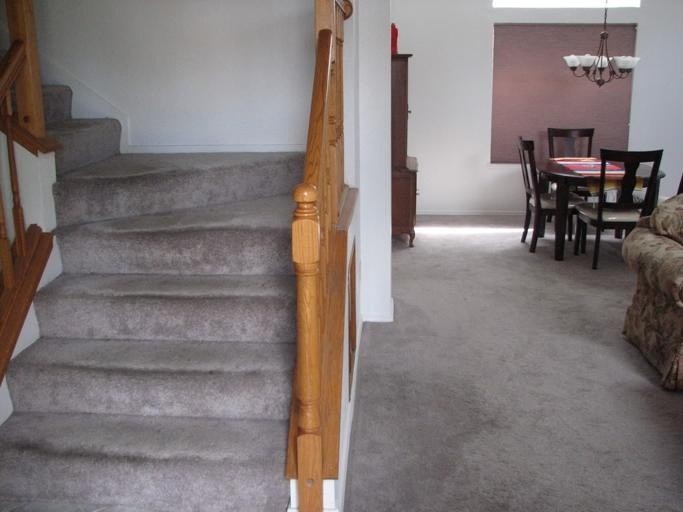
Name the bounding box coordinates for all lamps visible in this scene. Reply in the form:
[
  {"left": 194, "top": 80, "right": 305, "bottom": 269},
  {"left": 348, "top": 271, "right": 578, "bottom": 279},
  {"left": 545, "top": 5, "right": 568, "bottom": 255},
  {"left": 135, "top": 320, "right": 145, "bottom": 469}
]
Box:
[{"left": 562, "top": 0, "right": 640, "bottom": 88}]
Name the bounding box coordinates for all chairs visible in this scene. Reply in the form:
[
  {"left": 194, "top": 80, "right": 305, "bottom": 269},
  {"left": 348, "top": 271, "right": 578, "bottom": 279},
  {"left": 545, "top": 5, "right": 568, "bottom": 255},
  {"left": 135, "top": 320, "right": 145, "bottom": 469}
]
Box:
[
  {"left": 515, "top": 136, "right": 586, "bottom": 255},
  {"left": 547, "top": 128, "right": 604, "bottom": 232},
  {"left": 574, "top": 148, "right": 664, "bottom": 270}
]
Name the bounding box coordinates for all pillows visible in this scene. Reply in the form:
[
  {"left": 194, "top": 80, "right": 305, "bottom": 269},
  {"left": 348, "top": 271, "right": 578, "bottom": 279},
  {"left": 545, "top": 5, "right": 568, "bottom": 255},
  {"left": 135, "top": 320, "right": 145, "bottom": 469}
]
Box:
[{"left": 649, "top": 193, "right": 683, "bottom": 246}]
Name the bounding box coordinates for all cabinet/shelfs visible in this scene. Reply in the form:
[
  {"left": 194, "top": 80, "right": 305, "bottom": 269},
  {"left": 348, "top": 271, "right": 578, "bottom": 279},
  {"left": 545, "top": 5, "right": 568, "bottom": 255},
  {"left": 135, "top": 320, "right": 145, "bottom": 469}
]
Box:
[{"left": 391, "top": 53, "right": 418, "bottom": 248}]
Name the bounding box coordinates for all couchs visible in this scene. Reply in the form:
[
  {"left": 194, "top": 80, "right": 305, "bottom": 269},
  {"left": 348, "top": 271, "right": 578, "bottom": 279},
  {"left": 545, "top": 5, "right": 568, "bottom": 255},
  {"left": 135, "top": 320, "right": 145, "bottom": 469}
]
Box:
[{"left": 621, "top": 193, "right": 683, "bottom": 392}]
